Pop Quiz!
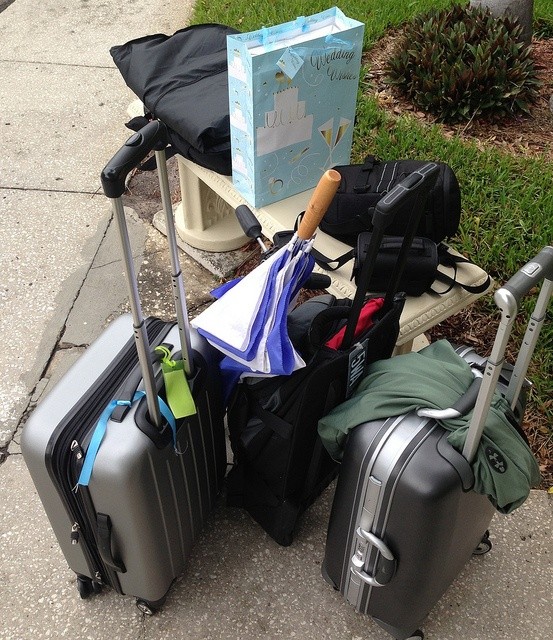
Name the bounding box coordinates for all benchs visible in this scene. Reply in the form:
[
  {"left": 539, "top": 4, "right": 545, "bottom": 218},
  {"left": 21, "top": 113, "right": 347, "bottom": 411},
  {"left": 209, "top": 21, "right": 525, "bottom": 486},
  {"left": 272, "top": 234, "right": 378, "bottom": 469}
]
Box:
[{"left": 125, "top": 97, "right": 495, "bottom": 355}]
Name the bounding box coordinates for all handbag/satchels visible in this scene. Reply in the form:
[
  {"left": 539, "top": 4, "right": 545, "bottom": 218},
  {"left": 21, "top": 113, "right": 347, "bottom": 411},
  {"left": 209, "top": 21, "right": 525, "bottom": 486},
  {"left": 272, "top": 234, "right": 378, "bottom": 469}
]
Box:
[
  {"left": 318, "top": 159, "right": 462, "bottom": 248},
  {"left": 357, "top": 235, "right": 442, "bottom": 297},
  {"left": 226, "top": 6, "right": 366, "bottom": 209}
]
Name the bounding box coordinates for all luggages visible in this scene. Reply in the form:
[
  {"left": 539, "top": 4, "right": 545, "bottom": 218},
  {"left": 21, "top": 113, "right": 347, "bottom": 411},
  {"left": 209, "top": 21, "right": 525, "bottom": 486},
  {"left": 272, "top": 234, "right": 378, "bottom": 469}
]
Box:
[
  {"left": 322, "top": 246, "right": 553, "bottom": 640},
  {"left": 227, "top": 161, "right": 439, "bottom": 545},
  {"left": 20, "top": 118, "right": 224, "bottom": 614}
]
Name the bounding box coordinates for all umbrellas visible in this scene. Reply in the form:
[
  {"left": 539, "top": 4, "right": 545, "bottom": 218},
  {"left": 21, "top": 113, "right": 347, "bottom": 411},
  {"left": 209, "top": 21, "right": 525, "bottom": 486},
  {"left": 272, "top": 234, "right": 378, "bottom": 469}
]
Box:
[{"left": 188, "top": 169, "right": 342, "bottom": 409}]
